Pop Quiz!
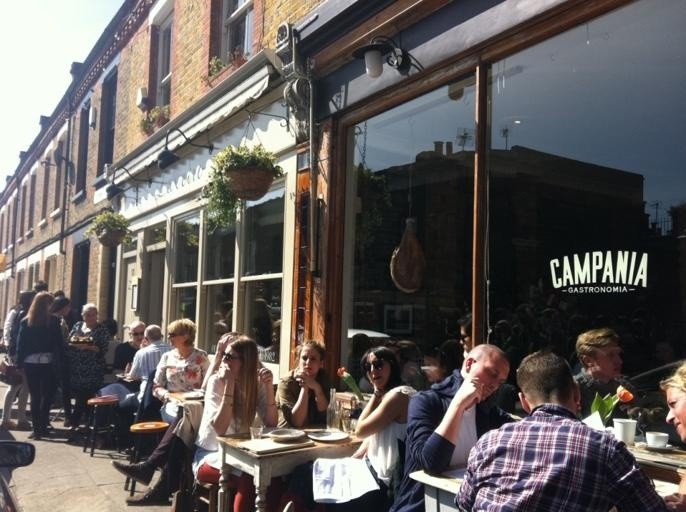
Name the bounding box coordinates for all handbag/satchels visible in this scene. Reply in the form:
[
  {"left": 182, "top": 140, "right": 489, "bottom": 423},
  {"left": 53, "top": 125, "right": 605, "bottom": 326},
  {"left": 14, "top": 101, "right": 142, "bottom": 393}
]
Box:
[{"left": 1, "top": 358, "right": 22, "bottom": 385}]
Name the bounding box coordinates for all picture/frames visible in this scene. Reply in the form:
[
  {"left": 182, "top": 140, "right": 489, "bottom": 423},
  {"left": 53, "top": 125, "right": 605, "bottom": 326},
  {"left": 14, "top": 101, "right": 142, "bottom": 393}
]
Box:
[
  {"left": 352, "top": 299, "right": 377, "bottom": 330},
  {"left": 382, "top": 303, "right": 416, "bottom": 335}
]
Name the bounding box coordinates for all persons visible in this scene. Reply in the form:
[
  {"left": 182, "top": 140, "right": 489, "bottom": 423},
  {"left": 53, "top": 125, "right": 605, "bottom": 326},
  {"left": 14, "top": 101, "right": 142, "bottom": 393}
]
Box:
[
  {"left": 97, "top": 319, "right": 210, "bottom": 463},
  {"left": 272, "top": 339, "right": 333, "bottom": 430},
  {"left": 279, "top": 346, "right": 417, "bottom": 512},
  {"left": 570, "top": 328, "right": 651, "bottom": 429},
  {"left": 111, "top": 331, "right": 279, "bottom": 512},
  {"left": 658, "top": 362, "right": 685, "bottom": 512},
  {"left": 347, "top": 281, "right": 606, "bottom": 390},
  {"left": 452, "top": 349, "right": 669, "bottom": 512},
  {"left": 181, "top": 281, "right": 280, "bottom": 364},
  {"left": 1, "top": 280, "right": 109, "bottom": 440},
  {"left": 388, "top": 344, "right": 516, "bottom": 512}
]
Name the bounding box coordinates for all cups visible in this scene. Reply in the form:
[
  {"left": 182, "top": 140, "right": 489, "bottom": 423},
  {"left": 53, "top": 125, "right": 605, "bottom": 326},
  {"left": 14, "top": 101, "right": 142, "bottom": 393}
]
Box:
[
  {"left": 249, "top": 424, "right": 263, "bottom": 445},
  {"left": 612, "top": 417, "right": 638, "bottom": 447}
]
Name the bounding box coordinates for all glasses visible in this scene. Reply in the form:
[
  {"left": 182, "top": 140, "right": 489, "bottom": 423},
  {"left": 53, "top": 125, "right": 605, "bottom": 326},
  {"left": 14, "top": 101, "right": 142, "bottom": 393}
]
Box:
[
  {"left": 364, "top": 362, "right": 383, "bottom": 371},
  {"left": 131, "top": 332, "right": 143, "bottom": 336},
  {"left": 169, "top": 333, "right": 177, "bottom": 337},
  {"left": 221, "top": 352, "right": 233, "bottom": 360}
]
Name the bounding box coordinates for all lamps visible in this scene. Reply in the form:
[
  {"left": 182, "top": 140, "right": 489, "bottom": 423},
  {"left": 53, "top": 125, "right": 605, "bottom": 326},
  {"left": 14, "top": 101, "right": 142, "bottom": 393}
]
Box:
[
  {"left": 104, "top": 166, "right": 151, "bottom": 202},
  {"left": 350, "top": 32, "right": 406, "bottom": 80},
  {"left": 155, "top": 126, "right": 213, "bottom": 170}
]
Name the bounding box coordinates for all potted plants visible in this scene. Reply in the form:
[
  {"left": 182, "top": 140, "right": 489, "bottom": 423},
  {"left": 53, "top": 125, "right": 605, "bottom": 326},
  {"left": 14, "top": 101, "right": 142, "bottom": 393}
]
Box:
[
  {"left": 204, "top": 142, "right": 284, "bottom": 234},
  {"left": 136, "top": 117, "right": 154, "bottom": 138},
  {"left": 146, "top": 102, "right": 169, "bottom": 128},
  {"left": 82, "top": 210, "right": 132, "bottom": 248}
]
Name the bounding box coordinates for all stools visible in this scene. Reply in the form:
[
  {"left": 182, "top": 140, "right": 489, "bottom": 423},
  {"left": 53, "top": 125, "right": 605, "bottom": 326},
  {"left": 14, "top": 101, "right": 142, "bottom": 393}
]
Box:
[
  {"left": 83, "top": 396, "right": 121, "bottom": 457},
  {"left": 192, "top": 478, "right": 219, "bottom": 512},
  {"left": 123, "top": 420, "right": 170, "bottom": 497}
]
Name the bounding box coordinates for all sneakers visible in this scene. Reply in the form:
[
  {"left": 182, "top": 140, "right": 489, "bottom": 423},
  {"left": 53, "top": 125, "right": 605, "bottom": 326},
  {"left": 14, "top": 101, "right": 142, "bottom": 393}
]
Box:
[
  {"left": 125, "top": 483, "right": 171, "bottom": 505},
  {"left": 111, "top": 460, "right": 155, "bottom": 485}
]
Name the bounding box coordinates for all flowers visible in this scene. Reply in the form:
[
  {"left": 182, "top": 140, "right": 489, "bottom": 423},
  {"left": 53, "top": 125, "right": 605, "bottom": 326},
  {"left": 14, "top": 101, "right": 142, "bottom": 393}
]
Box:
[
  {"left": 336, "top": 366, "right": 364, "bottom": 401},
  {"left": 590, "top": 385, "right": 634, "bottom": 427}
]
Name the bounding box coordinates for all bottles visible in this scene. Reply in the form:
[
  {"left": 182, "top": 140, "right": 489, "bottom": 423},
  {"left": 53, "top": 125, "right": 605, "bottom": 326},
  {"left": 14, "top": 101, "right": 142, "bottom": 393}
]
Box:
[{"left": 327, "top": 387, "right": 361, "bottom": 432}]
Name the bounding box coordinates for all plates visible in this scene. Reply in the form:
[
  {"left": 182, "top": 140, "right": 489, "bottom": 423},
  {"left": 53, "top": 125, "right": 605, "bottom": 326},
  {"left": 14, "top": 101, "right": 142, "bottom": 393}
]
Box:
[
  {"left": 646, "top": 444, "right": 678, "bottom": 452},
  {"left": 182, "top": 390, "right": 204, "bottom": 399},
  {"left": 307, "top": 431, "right": 349, "bottom": 441},
  {"left": 269, "top": 428, "right": 305, "bottom": 443}
]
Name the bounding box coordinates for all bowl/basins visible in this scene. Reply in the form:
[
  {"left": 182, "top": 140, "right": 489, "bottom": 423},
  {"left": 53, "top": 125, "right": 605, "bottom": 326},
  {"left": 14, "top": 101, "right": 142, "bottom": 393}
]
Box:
[{"left": 644, "top": 430, "right": 669, "bottom": 447}]
